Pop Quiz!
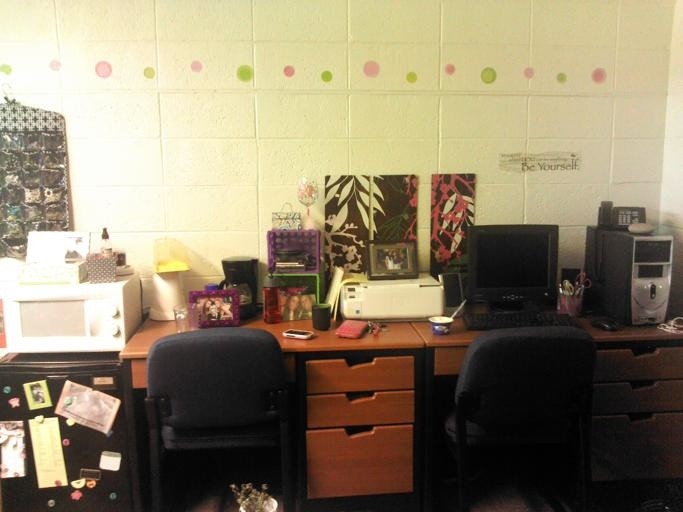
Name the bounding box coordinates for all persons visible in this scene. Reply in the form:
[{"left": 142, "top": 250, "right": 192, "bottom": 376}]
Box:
[
  {"left": 197, "top": 298, "right": 233, "bottom": 321},
  {"left": 281, "top": 296, "right": 315, "bottom": 321},
  {"left": 385, "top": 250, "right": 404, "bottom": 269}
]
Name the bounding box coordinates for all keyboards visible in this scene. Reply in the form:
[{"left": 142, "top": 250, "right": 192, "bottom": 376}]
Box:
[{"left": 462, "top": 313, "right": 582, "bottom": 330}]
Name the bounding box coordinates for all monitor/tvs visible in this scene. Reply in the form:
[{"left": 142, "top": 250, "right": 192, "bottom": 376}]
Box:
[{"left": 465, "top": 225, "right": 558, "bottom": 310}]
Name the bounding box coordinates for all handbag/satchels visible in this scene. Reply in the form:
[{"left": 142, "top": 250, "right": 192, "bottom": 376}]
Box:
[{"left": 270, "top": 201, "right": 302, "bottom": 231}]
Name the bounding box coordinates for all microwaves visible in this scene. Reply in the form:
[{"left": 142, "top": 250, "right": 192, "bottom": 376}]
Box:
[{"left": 4, "top": 274, "right": 141, "bottom": 353}]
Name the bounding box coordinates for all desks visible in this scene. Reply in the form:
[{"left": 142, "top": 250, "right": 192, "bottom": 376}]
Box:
[
  {"left": 122, "top": 309, "right": 425, "bottom": 501},
  {"left": 412, "top": 317, "right": 682, "bottom": 482}
]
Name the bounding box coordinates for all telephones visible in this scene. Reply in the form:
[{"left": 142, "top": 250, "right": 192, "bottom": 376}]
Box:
[{"left": 597, "top": 200, "right": 646, "bottom": 231}]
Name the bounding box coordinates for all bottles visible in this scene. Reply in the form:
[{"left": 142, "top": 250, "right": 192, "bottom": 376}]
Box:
[
  {"left": 98, "top": 227, "right": 113, "bottom": 260},
  {"left": 260, "top": 274, "right": 283, "bottom": 324}
]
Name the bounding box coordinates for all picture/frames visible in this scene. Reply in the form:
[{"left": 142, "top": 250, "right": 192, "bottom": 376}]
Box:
[
  {"left": 188, "top": 289, "right": 240, "bottom": 328},
  {"left": 367, "top": 240, "right": 419, "bottom": 280}
]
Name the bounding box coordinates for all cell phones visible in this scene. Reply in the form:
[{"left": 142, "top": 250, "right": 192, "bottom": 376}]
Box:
[{"left": 282, "top": 328, "right": 314, "bottom": 340}]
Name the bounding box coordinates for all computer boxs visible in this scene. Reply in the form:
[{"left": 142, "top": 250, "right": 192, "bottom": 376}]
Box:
[{"left": 583, "top": 226, "right": 674, "bottom": 325}]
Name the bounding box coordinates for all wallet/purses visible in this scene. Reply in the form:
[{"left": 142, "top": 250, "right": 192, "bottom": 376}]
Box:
[{"left": 335, "top": 319, "right": 369, "bottom": 339}]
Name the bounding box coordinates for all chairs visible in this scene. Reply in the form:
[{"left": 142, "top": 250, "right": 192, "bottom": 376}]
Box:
[
  {"left": 144, "top": 327, "right": 296, "bottom": 512},
  {"left": 441, "top": 326, "right": 596, "bottom": 512}
]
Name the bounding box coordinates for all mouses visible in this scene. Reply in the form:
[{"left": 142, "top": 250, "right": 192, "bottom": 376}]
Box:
[{"left": 591, "top": 319, "right": 619, "bottom": 331}]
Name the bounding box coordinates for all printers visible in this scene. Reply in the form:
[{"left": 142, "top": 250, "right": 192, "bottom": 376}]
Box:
[{"left": 340, "top": 272, "right": 445, "bottom": 322}]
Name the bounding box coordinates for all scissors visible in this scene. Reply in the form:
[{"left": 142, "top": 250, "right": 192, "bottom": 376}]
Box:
[{"left": 574, "top": 272, "right": 592, "bottom": 289}]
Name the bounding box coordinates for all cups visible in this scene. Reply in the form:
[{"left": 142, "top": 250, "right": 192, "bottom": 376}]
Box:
[
  {"left": 559, "top": 292, "right": 583, "bottom": 317},
  {"left": 428, "top": 316, "right": 454, "bottom": 336},
  {"left": 312, "top": 305, "right": 332, "bottom": 331},
  {"left": 173, "top": 302, "right": 204, "bottom": 332}
]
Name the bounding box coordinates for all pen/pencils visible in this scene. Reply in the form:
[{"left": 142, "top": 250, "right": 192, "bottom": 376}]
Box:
[{"left": 557, "top": 278, "right": 584, "bottom": 296}]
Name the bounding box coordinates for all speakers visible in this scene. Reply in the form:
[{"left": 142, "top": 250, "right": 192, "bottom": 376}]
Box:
[
  {"left": 561, "top": 268, "right": 582, "bottom": 288},
  {"left": 438, "top": 272, "right": 464, "bottom": 311}
]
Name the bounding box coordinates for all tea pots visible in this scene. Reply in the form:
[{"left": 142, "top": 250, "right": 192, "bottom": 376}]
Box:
[{"left": 218, "top": 255, "right": 259, "bottom": 319}]
